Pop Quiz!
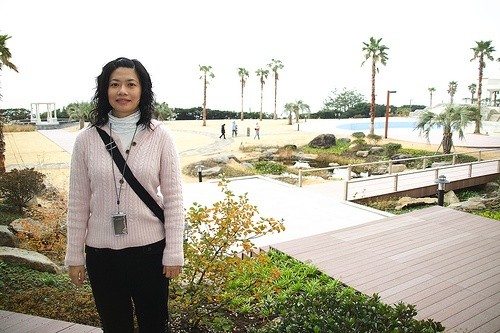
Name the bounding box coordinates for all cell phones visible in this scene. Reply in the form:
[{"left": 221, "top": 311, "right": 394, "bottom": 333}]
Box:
[{"left": 112, "top": 214, "right": 128, "bottom": 236}]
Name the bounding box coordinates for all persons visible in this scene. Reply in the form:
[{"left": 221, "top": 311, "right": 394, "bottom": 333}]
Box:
[
  {"left": 66, "top": 57, "right": 184, "bottom": 333},
  {"left": 232, "top": 121, "right": 237, "bottom": 137},
  {"left": 219, "top": 124, "right": 226, "bottom": 139},
  {"left": 254, "top": 123, "right": 260, "bottom": 139}
]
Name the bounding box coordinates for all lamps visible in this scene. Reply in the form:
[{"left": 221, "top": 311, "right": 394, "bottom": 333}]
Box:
[
  {"left": 195, "top": 161, "right": 204, "bottom": 172},
  {"left": 434, "top": 174, "right": 449, "bottom": 190}
]
